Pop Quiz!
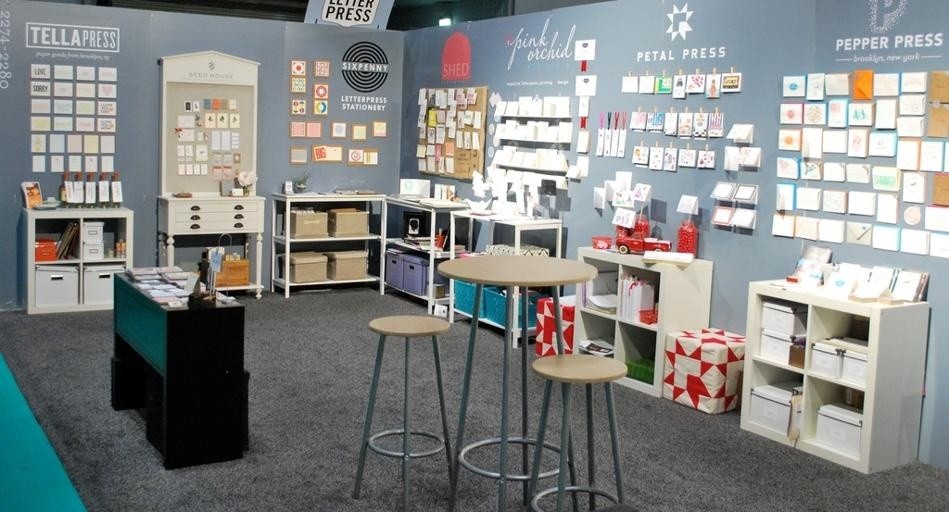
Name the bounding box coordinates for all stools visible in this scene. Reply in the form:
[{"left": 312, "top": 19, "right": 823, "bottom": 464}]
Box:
[
  {"left": 529, "top": 354, "right": 628, "bottom": 512},
  {"left": 662, "top": 326, "right": 746, "bottom": 414},
  {"left": 536, "top": 294, "right": 575, "bottom": 359},
  {"left": 351, "top": 315, "right": 452, "bottom": 512}
]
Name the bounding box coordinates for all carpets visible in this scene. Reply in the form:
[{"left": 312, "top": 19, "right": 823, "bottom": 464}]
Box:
[{"left": 0, "top": 278, "right": 949, "bottom": 511}]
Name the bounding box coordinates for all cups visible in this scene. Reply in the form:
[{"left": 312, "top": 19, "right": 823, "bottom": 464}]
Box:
[{"left": 435, "top": 235, "right": 444, "bottom": 247}]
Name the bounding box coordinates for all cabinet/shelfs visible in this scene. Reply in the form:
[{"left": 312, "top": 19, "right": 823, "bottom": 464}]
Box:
[
  {"left": 572, "top": 246, "right": 714, "bottom": 398},
  {"left": 387, "top": 197, "right": 470, "bottom": 315},
  {"left": 448, "top": 209, "right": 563, "bottom": 349},
  {"left": 111, "top": 265, "right": 249, "bottom": 471},
  {"left": 22, "top": 206, "right": 135, "bottom": 315},
  {"left": 157, "top": 50, "right": 266, "bottom": 299},
  {"left": 739, "top": 274, "right": 930, "bottom": 474},
  {"left": 271, "top": 192, "right": 388, "bottom": 298}
]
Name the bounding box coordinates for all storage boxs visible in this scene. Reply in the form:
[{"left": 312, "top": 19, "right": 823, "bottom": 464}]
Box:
[
  {"left": 454, "top": 279, "right": 550, "bottom": 328},
  {"left": 201, "top": 258, "right": 250, "bottom": 287},
  {"left": 386, "top": 251, "right": 449, "bottom": 296},
  {"left": 749, "top": 301, "right": 868, "bottom": 459},
  {"left": 36, "top": 222, "right": 124, "bottom": 307},
  {"left": 280, "top": 209, "right": 368, "bottom": 284}
]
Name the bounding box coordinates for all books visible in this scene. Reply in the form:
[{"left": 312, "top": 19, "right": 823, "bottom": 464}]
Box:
[
  {"left": 579, "top": 271, "right": 620, "bottom": 316},
  {"left": 404, "top": 236, "right": 438, "bottom": 247},
  {"left": 575, "top": 335, "right": 615, "bottom": 358},
  {"left": 124, "top": 264, "right": 236, "bottom": 309},
  {"left": 334, "top": 188, "right": 451, "bottom": 207},
  {"left": 641, "top": 250, "right": 695, "bottom": 267},
  {"left": 771, "top": 243, "right": 931, "bottom": 305}
]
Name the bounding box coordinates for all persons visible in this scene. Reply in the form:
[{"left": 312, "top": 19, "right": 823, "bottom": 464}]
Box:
[{"left": 409, "top": 219, "right": 419, "bottom": 235}]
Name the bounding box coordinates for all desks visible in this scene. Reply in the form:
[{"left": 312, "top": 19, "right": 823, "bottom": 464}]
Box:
[{"left": 437, "top": 255, "right": 599, "bottom": 512}]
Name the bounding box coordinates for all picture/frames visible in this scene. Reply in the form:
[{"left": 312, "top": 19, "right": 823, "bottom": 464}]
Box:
[{"left": 289, "top": 58, "right": 389, "bottom": 168}]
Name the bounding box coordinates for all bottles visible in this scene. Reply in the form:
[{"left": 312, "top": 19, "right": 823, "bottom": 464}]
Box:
[
  {"left": 59, "top": 168, "right": 123, "bottom": 210},
  {"left": 677, "top": 220, "right": 698, "bottom": 258},
  {"left": 592, "top": 212, "right": 649, "bottom": 250},
  {"left": 198, "top": 249, "right": 211, "bottom": 285}
]
